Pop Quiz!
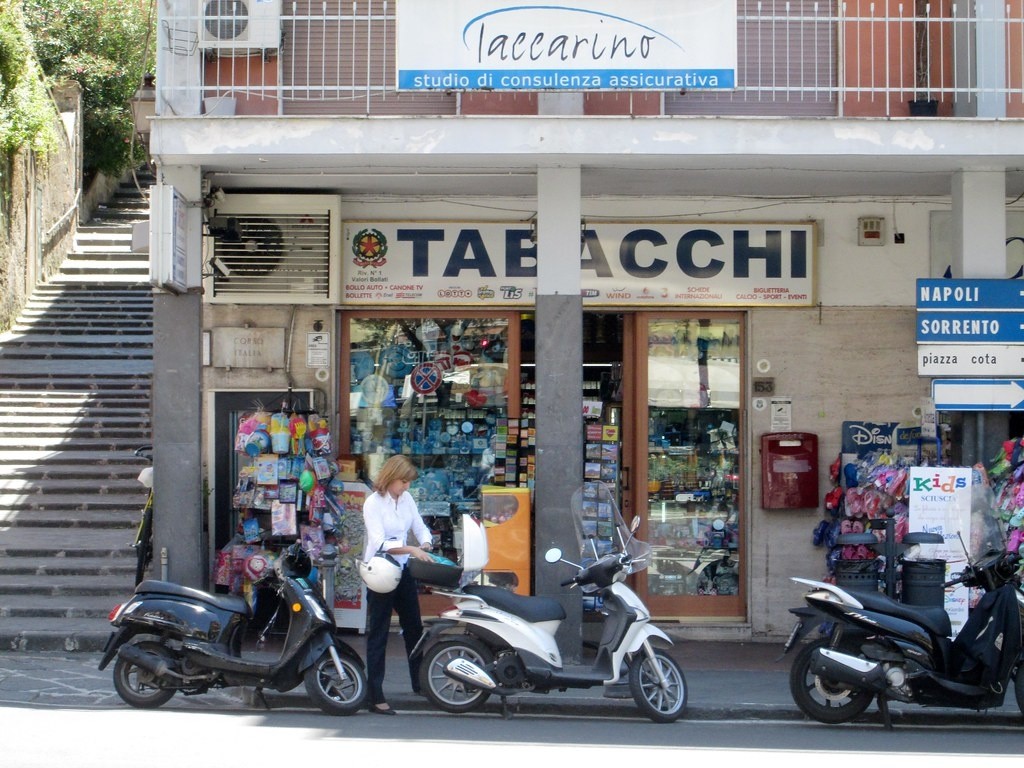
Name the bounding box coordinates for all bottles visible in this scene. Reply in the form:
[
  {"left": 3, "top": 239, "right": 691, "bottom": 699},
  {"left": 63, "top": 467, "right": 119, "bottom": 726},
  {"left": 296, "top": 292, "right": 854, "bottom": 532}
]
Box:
[
  {"left": 647, "top": 456, "right": 661, "bottom": 494},
  {"left": 678, "top": 481, "right": 685, "bottom": 491},
  {"left": 674, "top": 526, "right": 681, "bottom": 538}
]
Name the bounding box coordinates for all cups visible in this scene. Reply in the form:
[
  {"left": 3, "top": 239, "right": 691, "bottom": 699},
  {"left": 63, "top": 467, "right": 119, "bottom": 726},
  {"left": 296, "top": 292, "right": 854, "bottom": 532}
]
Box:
[
  {"left": 712, "top": 533, "right": 722, "bottom": 546},
  {"left": 731, "top": 534, "right": 738, "bottom": 543}
]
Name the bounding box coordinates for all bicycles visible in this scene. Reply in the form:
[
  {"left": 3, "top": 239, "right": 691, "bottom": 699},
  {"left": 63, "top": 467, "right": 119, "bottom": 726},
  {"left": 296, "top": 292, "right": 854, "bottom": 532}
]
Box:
[{"left": 127, "top": 445, "right": 152, "bottom": 587}]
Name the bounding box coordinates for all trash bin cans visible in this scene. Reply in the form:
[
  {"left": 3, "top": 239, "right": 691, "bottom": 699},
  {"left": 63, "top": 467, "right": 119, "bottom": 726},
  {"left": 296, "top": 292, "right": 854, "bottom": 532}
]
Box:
[
  {"left": 834, "top": 532, "right": 881, "bottom": 592},
  {"left": 899, "top": 532, "right": 947, "bottom": 607}
]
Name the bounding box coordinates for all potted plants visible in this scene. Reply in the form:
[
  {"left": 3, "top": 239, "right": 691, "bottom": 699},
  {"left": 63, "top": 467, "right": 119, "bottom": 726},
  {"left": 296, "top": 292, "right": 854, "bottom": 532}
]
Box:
[{"left": 907, "top": 0, "right": 940, "bottom": 117}]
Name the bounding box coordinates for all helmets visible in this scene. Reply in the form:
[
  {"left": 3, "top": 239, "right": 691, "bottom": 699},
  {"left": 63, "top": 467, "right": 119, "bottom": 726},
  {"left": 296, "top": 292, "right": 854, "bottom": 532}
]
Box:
[{"left": 353, "top": 555, "right": 407, "bottom": 595}]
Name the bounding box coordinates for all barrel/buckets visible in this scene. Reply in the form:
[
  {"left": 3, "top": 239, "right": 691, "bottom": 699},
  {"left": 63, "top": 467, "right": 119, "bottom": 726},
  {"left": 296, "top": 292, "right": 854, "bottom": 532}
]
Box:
[{"left": 234, "top": 414, "right": 331, "bottom": 460}]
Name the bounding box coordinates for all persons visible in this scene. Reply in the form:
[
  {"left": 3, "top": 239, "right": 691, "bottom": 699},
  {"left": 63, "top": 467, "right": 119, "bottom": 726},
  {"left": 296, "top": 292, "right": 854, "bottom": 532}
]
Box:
[{"left": 361, "top": 454, "right": 434, "bottom": 717}]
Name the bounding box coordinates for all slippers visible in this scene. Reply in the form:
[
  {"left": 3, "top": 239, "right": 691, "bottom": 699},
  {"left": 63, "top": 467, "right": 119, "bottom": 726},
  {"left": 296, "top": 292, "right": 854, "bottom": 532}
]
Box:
[{"left": 810, "top": 433, "right": 1024, "bottom": 600}]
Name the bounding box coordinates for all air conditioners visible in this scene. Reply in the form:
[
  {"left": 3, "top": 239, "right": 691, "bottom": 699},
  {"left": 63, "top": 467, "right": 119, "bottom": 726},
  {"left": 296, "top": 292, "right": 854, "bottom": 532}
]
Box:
[
  {"left": 203, "top": 193, "right": 342, "bottom": 306},
  {"left": 198, "top": 0, "right": 283, "bottom": 58}
]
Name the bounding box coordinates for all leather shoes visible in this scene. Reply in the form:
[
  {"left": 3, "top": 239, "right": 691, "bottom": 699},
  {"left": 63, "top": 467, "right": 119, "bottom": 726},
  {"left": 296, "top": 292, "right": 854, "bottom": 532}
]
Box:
[{"left": 368, "top": 699, "right": 397, "bottom": 716}]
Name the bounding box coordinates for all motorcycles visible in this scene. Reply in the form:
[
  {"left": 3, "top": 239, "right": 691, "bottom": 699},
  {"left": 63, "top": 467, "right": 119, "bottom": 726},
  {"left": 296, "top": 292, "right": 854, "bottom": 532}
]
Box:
[
  {"left": 688, "top": 519, "right": 739, "bottom": 597},
  {"left": 97, "top": 536, "right": 368, "bottom": 717},
  {"left": 762, "top": 462, "right": 1024, "bottom": 732},
  {"left": 405, "top": 481, "right": 689, "bottom": 723},
  {"left": 693, "top": 516, "right": 739, "bottom": 570}
]
